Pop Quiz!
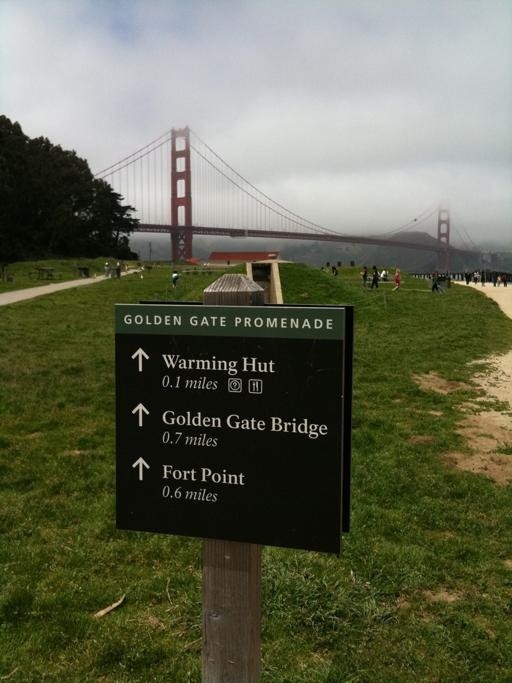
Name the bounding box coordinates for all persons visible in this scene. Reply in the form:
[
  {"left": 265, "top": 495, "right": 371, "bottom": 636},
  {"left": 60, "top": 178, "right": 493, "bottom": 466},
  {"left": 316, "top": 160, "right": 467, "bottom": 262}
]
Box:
[
  {"left": 103, "top": 260, "right": 110, "bottom": 278},
  {"left": 359, "top": 265, "right": 368, "bottom": 289},
  {"left": 465, "top": 271, "right": 470, "bottom": 284},
  {"left": 332, "top": 266, "right": 338, "bottom": 275},
  {"left": 369, "top": 264, "right": 379, "bottom": 288},
  {"left": 480, "top": 270, "right": 485, "bottom": 287},
  {"left": 377, "top": 268, "right": 389, "bottom": 280},
  {"left": 491, "top": 271, "right": 497, "bottom": 286},
  {"left": 473, "top": 272, "right": 477, "bottom": 284},
  {"left": 390, "top": 268, "right": 400, "bottom": 291},
  {"left": 496, "top": 273, "right": 501, "bottom": 287},
  {"left": 502, "top": 272, "right": 508, "bottom": 287},
  {"left": 171, "top": 269, "right": 178, "bottom": 289}
]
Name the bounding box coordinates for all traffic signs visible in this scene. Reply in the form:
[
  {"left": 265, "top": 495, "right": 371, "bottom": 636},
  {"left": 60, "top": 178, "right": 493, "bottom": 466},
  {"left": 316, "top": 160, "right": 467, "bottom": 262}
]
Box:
[{"left": 110, "top": 300, "right": 345, "bottom": 557}]
